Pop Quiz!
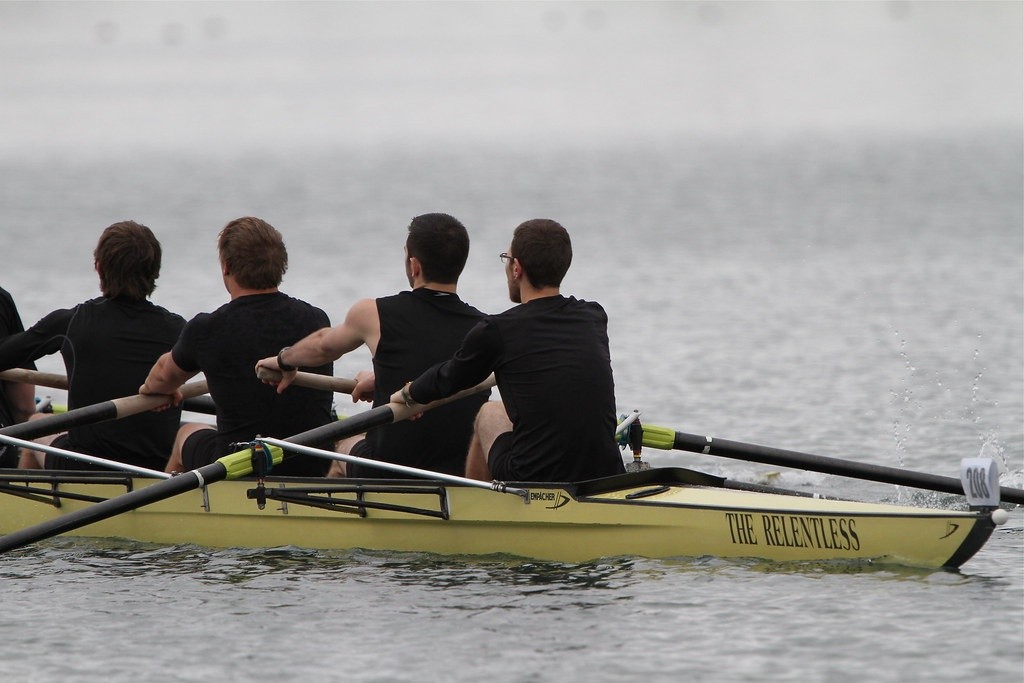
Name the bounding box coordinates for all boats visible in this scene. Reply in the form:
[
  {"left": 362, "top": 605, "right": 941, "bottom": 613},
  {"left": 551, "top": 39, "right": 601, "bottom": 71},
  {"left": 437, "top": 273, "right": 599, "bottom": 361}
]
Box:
[{"left": 0, "top": 485, "right": 1008, "bottom": 568}]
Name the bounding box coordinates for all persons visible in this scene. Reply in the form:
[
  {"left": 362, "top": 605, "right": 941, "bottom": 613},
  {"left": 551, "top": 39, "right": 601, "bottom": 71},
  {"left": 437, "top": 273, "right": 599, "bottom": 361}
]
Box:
[
  {"left": 254, "top": 213, "right": 495, "bottom": 481},
  {"left": 0, "top": 289, "right": 35, "bottom": 464},
  {"left": 391, "top": 220, "right": 624, "bottom": 483},
  {"left": 140, "top": 217, "right": 337, "bottom": 476},
  {"left": 0, "top": 220, "right": 188, "bottom": 474}
]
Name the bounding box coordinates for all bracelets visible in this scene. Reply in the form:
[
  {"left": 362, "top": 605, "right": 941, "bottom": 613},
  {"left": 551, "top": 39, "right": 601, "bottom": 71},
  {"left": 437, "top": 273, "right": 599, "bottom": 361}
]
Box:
[
  {"left": 402, "top": 382, "right": 417, "bottom": 405},
  {"left": 277, "top": 346, "right": 297, "bottom": 372}
]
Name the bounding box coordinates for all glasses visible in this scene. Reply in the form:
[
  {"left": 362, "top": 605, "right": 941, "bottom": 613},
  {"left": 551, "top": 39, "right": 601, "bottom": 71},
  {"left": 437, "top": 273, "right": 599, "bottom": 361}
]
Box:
[{"left": 500, "top": 253, "right": 524, "bottom": 270}]
